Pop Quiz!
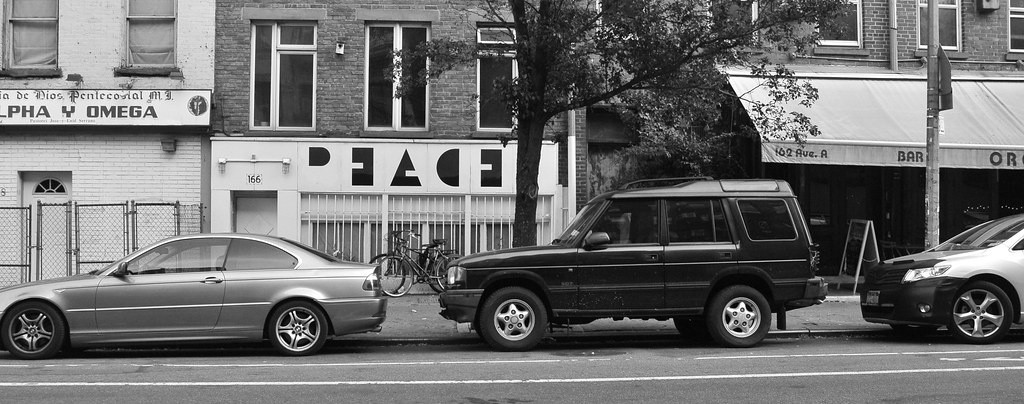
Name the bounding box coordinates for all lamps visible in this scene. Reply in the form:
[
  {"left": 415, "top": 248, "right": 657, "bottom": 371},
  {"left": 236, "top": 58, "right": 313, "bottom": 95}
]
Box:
[
  {"left": 168, "top": 72, "right": 185, "bottom": 89},
  {"left": 160, "top": 138, "right": 176, "bottom": 152},
  {"left": 66, "top": 74, "right": 83, "bottom": 89}
]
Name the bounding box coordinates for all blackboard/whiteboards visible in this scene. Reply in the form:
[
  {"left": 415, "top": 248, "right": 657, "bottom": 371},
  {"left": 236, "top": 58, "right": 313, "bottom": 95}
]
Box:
[{"left": 840, "top": 218, "right": 880, "bottom": 279}]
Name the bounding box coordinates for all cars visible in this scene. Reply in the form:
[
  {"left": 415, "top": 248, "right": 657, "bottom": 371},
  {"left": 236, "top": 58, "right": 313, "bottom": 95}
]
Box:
[
  {"left": 0, "top": 232, "right": 388, "bottom": 361},
  {"left": 859, "top": 213, "right": 1024, "bottom": 345}
]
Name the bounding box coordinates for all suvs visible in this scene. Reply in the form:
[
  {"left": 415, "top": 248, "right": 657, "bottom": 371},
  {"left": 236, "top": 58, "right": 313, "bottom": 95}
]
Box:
[{"left": 438, "top": 174, "right": 829, "bottom": 352}]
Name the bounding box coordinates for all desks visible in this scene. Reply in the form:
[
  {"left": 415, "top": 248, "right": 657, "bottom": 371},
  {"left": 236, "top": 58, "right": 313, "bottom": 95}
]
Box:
[{"left": 894, "top": 244, "right": 924, "bottom": 257}]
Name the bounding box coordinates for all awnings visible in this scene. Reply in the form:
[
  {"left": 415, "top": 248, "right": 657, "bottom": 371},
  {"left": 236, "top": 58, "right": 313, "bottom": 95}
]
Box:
[{"left": 726, "top": 76, "right": 1024, "bottom": 170}]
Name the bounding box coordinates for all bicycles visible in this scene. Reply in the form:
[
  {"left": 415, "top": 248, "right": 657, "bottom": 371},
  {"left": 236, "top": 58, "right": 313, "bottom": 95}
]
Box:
[{"left": 369, "top": 228, "right": 464, "bottom": 298}]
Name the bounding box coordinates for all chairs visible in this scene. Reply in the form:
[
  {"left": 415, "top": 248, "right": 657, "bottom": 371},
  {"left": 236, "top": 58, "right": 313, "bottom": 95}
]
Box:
[{"left": 879, "top": 240, "right": 896, "bottom": 260}]
